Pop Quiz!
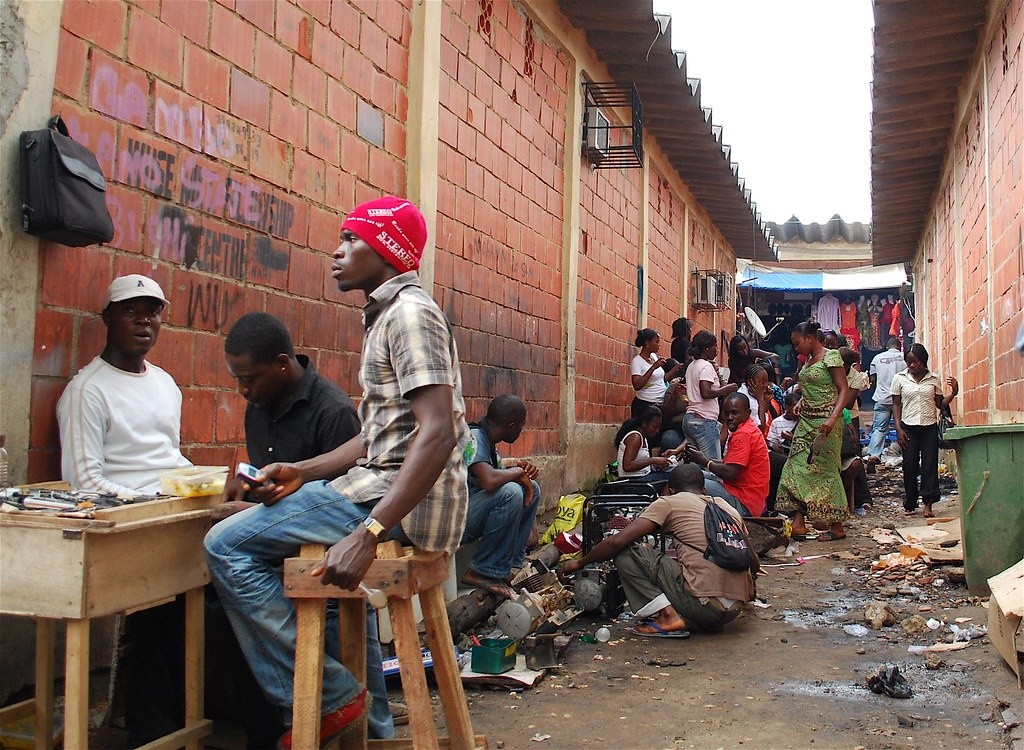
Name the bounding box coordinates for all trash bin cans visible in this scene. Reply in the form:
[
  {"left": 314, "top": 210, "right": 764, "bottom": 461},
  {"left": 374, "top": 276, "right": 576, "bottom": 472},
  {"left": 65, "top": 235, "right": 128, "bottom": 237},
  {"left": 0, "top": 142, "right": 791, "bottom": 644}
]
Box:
[{"left": 941, "top": 423, "right": 1024, "bottom": 601}]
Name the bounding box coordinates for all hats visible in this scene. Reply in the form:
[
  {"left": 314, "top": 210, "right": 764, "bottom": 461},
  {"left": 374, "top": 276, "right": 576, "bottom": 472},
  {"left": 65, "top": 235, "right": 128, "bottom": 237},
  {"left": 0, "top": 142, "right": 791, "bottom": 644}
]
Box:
[
  {"left": 103, "top": 274, "right": 171, "bottom": 311},
  {"left": 342, "top": 195, "right": 428, "bottom": 273}
]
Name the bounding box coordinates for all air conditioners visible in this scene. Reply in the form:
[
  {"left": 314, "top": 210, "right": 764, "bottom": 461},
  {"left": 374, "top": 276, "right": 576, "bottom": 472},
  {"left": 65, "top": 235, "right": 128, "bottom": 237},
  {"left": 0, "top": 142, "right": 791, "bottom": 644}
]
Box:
[
  {"left": 584, "top": 106, "right": 612, "bottom": 159},
  {"left": 694, "top": 277, "right": 718, "bottom": 308},
  {"left": 717, "top": 274, "right": 733, "bottom": 303}
]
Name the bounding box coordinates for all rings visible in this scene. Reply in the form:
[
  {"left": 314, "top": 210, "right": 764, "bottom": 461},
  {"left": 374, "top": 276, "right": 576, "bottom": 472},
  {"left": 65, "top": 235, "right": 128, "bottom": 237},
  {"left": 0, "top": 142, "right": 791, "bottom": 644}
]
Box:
[{"left": 754, "top": 385, "right": 757, "bottom": 389}]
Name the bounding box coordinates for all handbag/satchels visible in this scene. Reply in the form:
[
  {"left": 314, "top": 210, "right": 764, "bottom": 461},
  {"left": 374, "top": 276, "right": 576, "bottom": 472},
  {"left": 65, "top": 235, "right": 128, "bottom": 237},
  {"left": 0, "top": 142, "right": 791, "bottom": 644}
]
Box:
[
  {"left": 937, "top": 396, "right": 957, "bottom": 450},
  {"left": 18, "top": 115, "right": 114, "bottom": 247},
  {"left": 538, "top": 492, "right": 594, "bottom": 547}
]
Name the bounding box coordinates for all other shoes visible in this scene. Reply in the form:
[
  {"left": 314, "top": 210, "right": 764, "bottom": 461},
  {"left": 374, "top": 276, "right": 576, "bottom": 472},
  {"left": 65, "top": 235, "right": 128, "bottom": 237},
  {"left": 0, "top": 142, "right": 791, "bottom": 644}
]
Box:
[
  {"left": 807, "top": 433, "right": 827, "bottom": 464},
  {"left": 866, "top": 457, "right": 876, "bottom": 475}
]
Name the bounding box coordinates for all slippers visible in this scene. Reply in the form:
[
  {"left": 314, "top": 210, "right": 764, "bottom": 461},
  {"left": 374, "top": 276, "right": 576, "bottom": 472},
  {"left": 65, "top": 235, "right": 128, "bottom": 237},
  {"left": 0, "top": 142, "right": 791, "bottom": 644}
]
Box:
[
  {"left": 924, "top": 506, "right": 935, "bottom": 518},
  {"left": 791, "top": 535, "right": 807, "bottom": 541},
  {"left": 904, "top": 508, "right": 917, "bottom": 515},
  {"left": 460, "top": 576, "right": 512, "bottom": 598},
  {"left": 625, "top": 619, "right": 690, "bottom": 637},
  {"left": 817, "top": 530, "right": 846, "bottom": 542}
]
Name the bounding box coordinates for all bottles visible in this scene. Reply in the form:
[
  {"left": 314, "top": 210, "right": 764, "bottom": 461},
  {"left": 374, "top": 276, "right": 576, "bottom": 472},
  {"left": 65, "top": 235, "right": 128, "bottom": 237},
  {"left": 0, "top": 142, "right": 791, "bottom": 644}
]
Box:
[{"left": 0, "top": 435, "right": 9, "bottom": 491}]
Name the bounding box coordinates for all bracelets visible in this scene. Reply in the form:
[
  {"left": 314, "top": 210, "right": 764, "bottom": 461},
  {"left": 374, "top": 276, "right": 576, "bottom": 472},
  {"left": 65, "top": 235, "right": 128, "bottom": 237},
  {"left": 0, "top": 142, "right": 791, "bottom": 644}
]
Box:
[
  {"left": 952, "top": 392, "right": 958, "bottom": 396},
  {"left": 707, "top": 461, "right": 714, "bottom": 470},
  {"left": 780, "top": 385, "right": 785, "bottom": 389}
]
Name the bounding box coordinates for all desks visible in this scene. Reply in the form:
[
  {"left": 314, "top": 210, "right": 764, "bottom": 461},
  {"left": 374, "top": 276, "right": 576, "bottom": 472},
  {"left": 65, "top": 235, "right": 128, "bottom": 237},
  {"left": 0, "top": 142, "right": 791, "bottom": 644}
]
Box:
[{"left": 0, "top": 483, "right": 232, "bottom": 750}]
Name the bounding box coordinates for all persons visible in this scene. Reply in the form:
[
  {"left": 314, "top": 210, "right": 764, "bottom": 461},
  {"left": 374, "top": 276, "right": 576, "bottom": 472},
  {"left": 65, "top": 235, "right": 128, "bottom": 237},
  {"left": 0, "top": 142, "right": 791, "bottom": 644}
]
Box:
[
  {"left": 56, "top": 273, "right": 368, "bottom": 750},
  {"left": 557, "top": 464, "right": 759, "bottom": 639},
  {"left": 817, "top": 330, "right": 872, "bottom": 512},
  {"left": 891, "top": 343, "right": 958, "bottom": 519},
  {"left": 614, "top": 405, "right": 673, "bottom": 496},
  {"left": 460, "top": 395, "right": 540, "bottom": 598},
  {"left": 661, "top": 393, "right": 771, "bottom": 518},
  {"left": 204, "top": 196, "right": 470, "bottom": 750},
  {"left": 854, "top": 294, "right": 898, "bottom": 349},
  {"left": 774, "top": 322, "right": 850, "bottom": 541},
  {"left": 866, "top": 338, "right": 910, "bottom": 474},
  {"left": 630, "top": 317, "right": 873, "bottom": 510}
]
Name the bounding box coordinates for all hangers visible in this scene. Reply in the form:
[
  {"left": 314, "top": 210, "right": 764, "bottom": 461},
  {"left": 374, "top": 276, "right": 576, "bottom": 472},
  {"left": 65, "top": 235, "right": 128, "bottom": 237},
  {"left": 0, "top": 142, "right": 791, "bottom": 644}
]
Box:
[
  {"left": 820, "top": 291, "right": 838, "bottom": 300},
  {"left": 842, "top": 296, "right": 854, "bottom": 305}
]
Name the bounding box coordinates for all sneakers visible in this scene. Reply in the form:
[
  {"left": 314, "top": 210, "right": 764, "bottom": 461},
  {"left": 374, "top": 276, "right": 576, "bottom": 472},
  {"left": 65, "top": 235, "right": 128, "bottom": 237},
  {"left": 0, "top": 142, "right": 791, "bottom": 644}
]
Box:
[{"left": 276, "top": 683, "right": 373, "bottom": 750}]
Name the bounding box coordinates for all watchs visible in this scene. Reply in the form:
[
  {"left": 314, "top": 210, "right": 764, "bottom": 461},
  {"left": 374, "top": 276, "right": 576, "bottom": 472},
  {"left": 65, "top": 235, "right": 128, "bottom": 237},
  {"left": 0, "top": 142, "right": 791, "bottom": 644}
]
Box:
[{"left": 363, "top": 516, "right": 386, "bottom": 538}]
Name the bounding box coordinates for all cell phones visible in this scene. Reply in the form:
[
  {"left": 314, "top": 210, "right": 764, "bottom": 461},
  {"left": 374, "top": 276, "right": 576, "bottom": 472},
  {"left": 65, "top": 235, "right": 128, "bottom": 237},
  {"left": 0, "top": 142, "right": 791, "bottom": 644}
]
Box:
[
  {"left": 234, "top": 461, "right": 275, "bottom": 488},
  {"left": 23, "top": 496, "right": 76, "bottom": 509}
]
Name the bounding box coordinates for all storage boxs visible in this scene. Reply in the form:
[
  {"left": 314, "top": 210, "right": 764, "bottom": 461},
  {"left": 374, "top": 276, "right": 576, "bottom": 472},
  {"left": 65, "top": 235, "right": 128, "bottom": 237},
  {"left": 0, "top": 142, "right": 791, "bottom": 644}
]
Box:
[
  {"left": 468, "top": 636, "right": 519, "bottom": 673},
  {"left": 160, "top": 461, "right": 234, "bottom": 498}
]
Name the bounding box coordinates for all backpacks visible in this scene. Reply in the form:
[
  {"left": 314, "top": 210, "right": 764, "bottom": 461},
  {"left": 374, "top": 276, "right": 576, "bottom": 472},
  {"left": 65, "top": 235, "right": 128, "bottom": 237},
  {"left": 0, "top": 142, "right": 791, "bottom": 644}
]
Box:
[{"left": 670, "top": 494, "right": 752, "bottom": 571}]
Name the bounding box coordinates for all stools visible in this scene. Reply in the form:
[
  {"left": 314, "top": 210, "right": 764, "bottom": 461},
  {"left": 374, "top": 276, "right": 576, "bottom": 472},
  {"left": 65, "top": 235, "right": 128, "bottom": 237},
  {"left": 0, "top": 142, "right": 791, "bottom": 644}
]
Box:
[{"left": 283, "top": 540, "right": 487, "bottom": 750}]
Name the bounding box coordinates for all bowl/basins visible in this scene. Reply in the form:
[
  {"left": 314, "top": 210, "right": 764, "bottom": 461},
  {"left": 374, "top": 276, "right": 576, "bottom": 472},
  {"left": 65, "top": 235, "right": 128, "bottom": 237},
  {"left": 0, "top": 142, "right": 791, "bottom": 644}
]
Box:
[{"left": 158, "top": 466, "right": 230, "bottom": 497}]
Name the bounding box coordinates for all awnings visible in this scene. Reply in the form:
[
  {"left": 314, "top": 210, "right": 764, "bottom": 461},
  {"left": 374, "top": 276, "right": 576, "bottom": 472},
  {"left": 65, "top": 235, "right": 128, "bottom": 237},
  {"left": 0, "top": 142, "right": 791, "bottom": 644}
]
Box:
[{"left": 733, "top": 257, "right": 911, "bottom": 291}]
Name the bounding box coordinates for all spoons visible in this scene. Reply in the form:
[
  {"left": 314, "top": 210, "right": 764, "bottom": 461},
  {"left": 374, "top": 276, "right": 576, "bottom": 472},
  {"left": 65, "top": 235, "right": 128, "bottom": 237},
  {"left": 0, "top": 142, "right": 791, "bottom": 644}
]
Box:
[{"left": 358, "top": 581, "right": 388, "bottom": 608}]
